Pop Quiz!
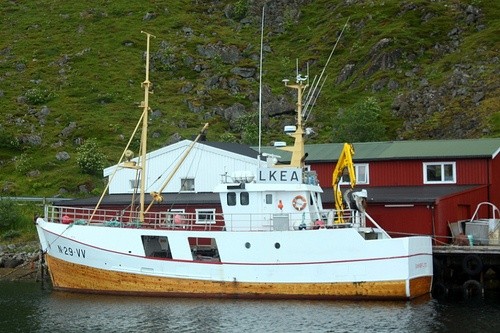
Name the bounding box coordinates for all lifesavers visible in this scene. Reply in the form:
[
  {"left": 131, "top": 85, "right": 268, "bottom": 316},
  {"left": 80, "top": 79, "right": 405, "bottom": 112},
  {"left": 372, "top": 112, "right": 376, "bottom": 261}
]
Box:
[{"left": 292, "top": 195, "right": 307, "bottom": 211}]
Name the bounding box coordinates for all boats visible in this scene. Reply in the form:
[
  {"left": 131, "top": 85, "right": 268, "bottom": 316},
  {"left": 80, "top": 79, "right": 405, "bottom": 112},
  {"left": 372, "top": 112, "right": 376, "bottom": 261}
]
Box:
[{"left": 35, "top": 27, "right": 437, "bottom": 302}]
